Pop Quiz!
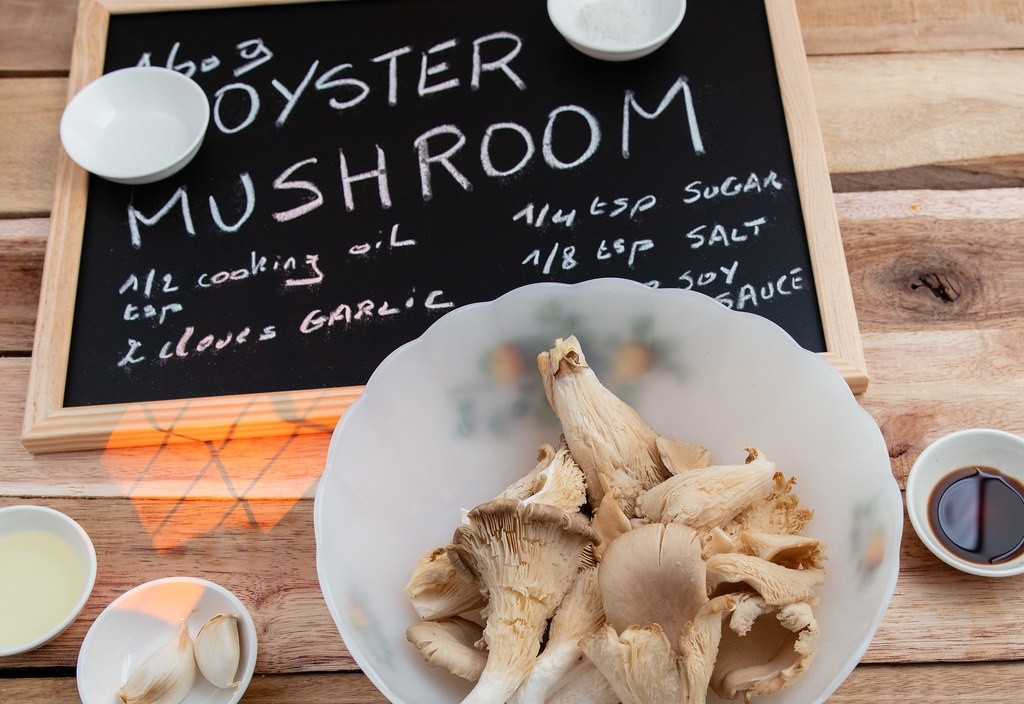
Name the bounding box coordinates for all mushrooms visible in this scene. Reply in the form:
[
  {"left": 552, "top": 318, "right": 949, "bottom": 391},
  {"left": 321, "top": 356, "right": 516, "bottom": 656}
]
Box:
[{"left": 407, "top": 334, "right": 830, "bottom": 704}]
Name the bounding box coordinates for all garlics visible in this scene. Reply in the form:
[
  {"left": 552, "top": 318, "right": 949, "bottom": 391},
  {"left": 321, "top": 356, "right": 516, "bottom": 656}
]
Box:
[{"left": 117, "top": 612, "right": 242, "bottom": 704}]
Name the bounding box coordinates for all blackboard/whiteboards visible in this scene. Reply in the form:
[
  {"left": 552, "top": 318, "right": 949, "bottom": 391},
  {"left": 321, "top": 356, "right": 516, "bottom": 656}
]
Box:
[{"left": 21, "top": 0, "right": 869, "bottom": 456}]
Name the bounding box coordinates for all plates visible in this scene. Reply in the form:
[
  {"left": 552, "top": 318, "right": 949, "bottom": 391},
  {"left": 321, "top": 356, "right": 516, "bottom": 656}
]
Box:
[
  {"left": 314, "top": 278, "right": 905, "bottom": 704},
  {"left": 59, "top": 66, "right": 211, "bottom": 186},
  {"left": 904, "top": 427, "right": 1024, "bottom": 579},
  {"left": 75, "top": 577, "right": 256, "bottom": 704},
  {"left": 547, "top": 1, "right": 686, "bottom": 61},
  {"left": 0, "top": 505, "right": 96, "bottom": 656}
]
